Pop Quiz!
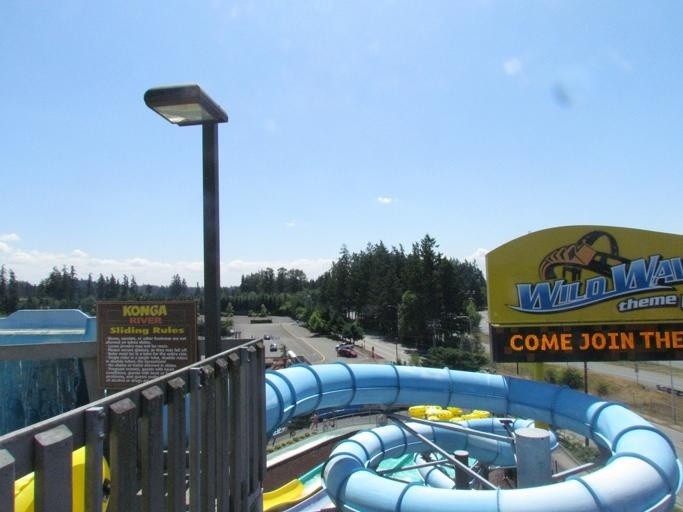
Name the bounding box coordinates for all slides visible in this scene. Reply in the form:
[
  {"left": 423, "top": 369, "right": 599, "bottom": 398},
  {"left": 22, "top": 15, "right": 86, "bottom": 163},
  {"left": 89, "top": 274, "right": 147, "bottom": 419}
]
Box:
[{"left": 263, "top": 463, "right": 322, "bottom": 511}]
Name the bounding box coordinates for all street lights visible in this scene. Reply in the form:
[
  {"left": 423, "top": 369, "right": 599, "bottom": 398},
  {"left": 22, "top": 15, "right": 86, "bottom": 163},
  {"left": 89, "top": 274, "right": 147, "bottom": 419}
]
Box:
[{"left": 143, "top": 83, "right": 228, "bottom": 359}]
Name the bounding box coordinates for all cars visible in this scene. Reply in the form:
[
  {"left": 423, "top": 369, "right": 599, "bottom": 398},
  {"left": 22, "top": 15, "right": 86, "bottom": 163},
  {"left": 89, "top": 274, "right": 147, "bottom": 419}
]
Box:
[
  {"left": 336, "top": 341, "right": 357, "bottom": 358},
  {"left": 263, "top": 334, "right": 269, "bottom": 340},
  {"left": 269, "top": 343, "right": 277, "bottom": 352}
]
